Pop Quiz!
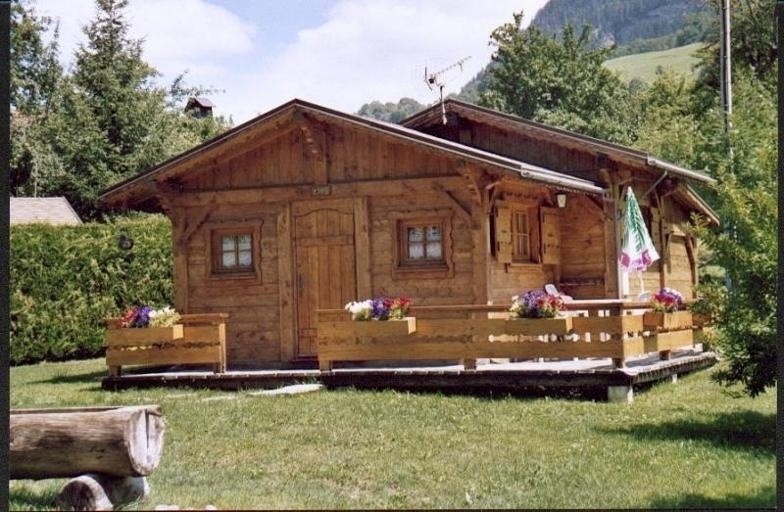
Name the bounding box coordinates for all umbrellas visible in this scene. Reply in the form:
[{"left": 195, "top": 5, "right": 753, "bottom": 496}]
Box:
[{"left": 618, "top": 185, "right": 662, "bottom": 302}]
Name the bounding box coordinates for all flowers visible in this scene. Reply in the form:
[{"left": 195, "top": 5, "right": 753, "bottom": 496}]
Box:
[
  {"left": 118, "top": 307, "right": 180, "bottom": 328},
  {"left": 650, "top": 289, "right": 684, "bottom": 311},
  {"left": 344, "top": 297, "right": 411, "bottom": 320},
  {"left": 511, "top": 290, "right": 562, "bottom": 319}
]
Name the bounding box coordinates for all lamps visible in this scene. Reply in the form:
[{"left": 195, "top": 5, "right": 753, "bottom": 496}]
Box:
[{"left": 556, "top": 190, "right": 568, "bottom": 209}]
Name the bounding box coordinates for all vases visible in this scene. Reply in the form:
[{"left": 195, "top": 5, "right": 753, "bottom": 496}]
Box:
[
  {"left": 104, "top": 324, "right": 183, "bottom": 344},
  {"left": 506, "top": 318, "right": 572, "bottom": 335},
  {"left": 342, "top": 317, "right": 415, "bottom": 337},
  {"left": 643, "top": 312, "right": 692, "bottom": 329}
]
